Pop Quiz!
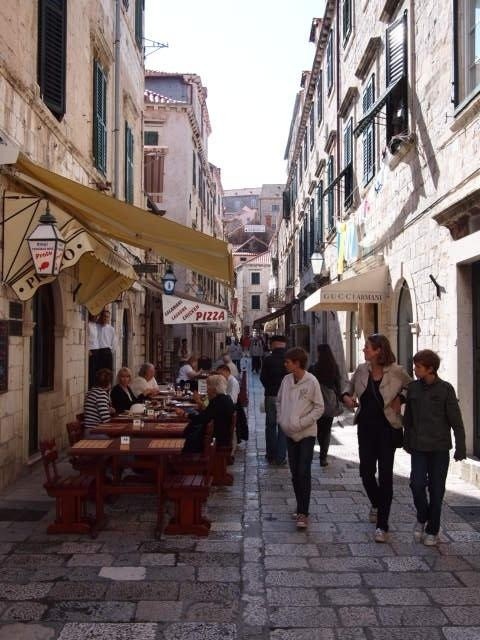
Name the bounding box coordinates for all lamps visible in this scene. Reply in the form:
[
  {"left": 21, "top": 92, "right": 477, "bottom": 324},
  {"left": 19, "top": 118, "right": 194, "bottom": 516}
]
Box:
[
  {"left": 310, "top": 240, "right": 340, "bottom": 274},
  {"left": 1, "top": 194, "right": 65, "bottom": 277},
  {"left": 154, "top": 261, "right": 178, "bottom": 296}
]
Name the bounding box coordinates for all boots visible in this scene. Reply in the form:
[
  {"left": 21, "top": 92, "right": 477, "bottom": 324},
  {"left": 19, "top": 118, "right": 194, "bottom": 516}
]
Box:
[{"left": 320, "top": 444, "right": 330, "bottom": 466}]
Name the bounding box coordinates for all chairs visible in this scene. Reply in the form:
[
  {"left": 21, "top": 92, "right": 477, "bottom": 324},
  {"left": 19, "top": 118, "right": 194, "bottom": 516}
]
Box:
[
  {"left": 37, "top": 413, "right": 123, "bottom": 535},
  {"left": 163, "top": 409, "right": 239, "bottom": 537},
  {"left": 239, "top": 373, "right": 248, "bottom": 406}
]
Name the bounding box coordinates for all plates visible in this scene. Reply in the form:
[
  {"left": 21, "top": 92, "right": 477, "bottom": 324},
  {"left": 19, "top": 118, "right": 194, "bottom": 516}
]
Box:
[{"left": 111, "top": 382, "right": 209, "bottom": 422}]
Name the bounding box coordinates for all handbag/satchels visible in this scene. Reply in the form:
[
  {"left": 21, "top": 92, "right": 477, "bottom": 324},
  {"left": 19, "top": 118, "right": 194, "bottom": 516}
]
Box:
[{"left": 320, "top": 383, "right": 344, "bottom": 417}]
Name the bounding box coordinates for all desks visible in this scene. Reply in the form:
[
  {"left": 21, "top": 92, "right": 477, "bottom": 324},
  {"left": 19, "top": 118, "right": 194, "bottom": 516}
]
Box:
[{"left": 71, "top": 392, "right": 205, "bottom": 541}]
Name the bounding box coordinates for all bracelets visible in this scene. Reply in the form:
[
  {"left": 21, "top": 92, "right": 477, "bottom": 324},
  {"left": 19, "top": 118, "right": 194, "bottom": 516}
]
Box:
[{"left": 182, "top": 412, "right": 185, "bottom": 418}]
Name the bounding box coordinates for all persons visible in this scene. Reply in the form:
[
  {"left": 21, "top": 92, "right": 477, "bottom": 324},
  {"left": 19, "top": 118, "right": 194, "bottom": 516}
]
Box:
[
  {"left": 98, "top": 310, "right": 116, "bottom": 387},
  {"left": 341, "top": 334, "right": 414, "bottom": 543},
  {"left": 175, "top": 332, "right": 270, "bottom": 404},
  {"left": 131, "top": 363, "right": 159, "bottom": 399},
  {"left": 403, "top": 349, "right": 466, "bottom": 546},
  {"left": 175, "top": 375, "right": 235, "bottom": 457},
  {"left": 260, "top": 335, "right": 290, "bottom": 466},
  {"left": 276, "top": 347, "right": 325, "bottom": 528},
  {"left": 83, "top": 368, "right": 116, "bottom": 440},
  {"left": 110, "top": 367, "right": 153, "bottom": 414},
  {"left": 88, "top": 310, "right": 100, "bottom": 391},
  {"left": 307, "top": 343, "right": 344, "bottom": 467}
]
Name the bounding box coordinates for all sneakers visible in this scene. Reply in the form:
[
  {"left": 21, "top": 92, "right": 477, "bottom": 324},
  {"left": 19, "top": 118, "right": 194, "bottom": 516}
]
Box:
[
  {"left": 423, "top": 533, "right": 438, "bottom": 545},
  {"left": 413, "top": 520, "right": 425, "bottom": 538},
  {"left": 375, "top": 528, "right": 385, "bottom": 542},
  {"left": 369, "top": 506, "right": 378, "bottom": 523},
  {"left": 292, "top": 508, "right": 309, "bottom": 528}
]
